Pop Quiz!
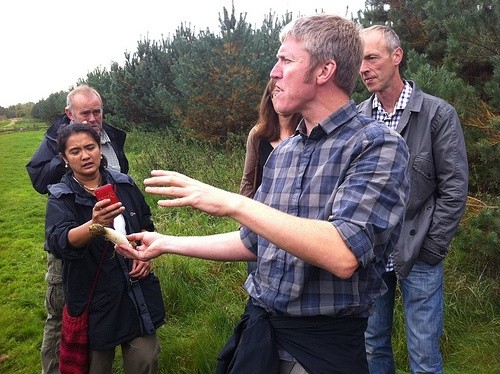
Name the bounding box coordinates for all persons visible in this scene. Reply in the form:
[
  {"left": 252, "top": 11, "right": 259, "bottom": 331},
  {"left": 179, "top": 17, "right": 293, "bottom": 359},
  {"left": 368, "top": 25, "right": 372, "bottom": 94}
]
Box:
[
  {"left": 114, "top": 14, "right": 412, "bottom": 374},
  {"left": 239, "top": 78, "right": 304, "bottom": 199},
  {"left": 44, "top": 122, "right": 166, "bottom": 374},
  {"left": 26, "top": 84, "right": 130, "bottom": 374},
  {"left": 353, "top": 24, "right": 468, "bottom": 374}
]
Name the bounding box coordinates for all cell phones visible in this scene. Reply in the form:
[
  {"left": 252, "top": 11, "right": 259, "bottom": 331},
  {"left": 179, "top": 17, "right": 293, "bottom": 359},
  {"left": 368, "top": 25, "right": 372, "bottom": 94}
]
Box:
[{"left": 94, "top": 184, "right": 120, "bottom": 210}]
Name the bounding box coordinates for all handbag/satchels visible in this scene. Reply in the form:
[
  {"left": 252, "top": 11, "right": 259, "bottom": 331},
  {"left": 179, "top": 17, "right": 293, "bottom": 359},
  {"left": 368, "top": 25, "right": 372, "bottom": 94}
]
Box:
[{"left": 59, "top": 302, "right": 89, "bottom": 374}]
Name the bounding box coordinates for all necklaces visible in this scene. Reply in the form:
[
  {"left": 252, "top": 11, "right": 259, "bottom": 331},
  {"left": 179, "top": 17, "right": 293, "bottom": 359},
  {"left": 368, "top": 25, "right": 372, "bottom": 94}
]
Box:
[{"left": 71, "top": 172, "right": 103, "bottom": 191}]
